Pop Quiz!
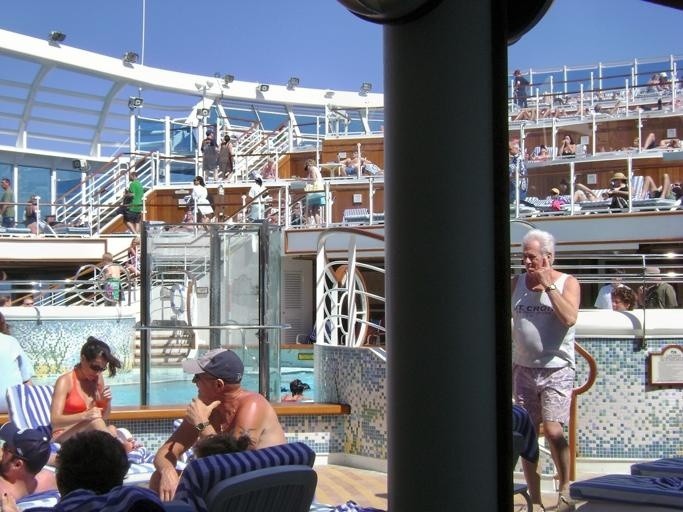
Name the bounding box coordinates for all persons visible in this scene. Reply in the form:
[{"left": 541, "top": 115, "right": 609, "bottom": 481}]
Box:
[
  {"left": 149, "top": 345, "right": 289, "bottom": 502},
  {"left": 98, "top": 252, "right": 128, "bottom": 306},
  {"left": 122, "top": 173, "right": 144, "bottom": 235},
  {"left": 0, "top": 177, "right": 80, "bottom": 236},
  {"left": 610, "top": 286, "right": 635, "bottom": 311},
  {"left": 55, "top": 429, "right": 130, "bottom": 498},
  {"left": 47, "top": 335, "right": 144, "bottom": 452},
  {"left": 508, "top": 68, "right": 682, "bottom": 220},
  {"left": 0, "top": 311, "right": 35, "bottom": 416},
  {"left": 20, "top": 295, "right": 34, "bottom": 306},
  {"left": 190, "top": 433, "right": 249, "bottom": 461},
  {"left": 594, "top": 265, "right": 639, "bottom": 310},
  {"left": 124, "top": 235, "right": 140, "bottom": 278},
  {"left": 280, "top": 378, "right": 310, "bottom": 401},
  {"left": 0, "top": 296, "right": 12, "bottom": 307},
  {"left": 180, "top": 129, "right": 373, "bottom": 230},
  {"left": 510, "top": 228, "right": 583, "bottom": 512},
  {"left": 634, "top": 267, "right": 678, "bottom": 309},
  {"left": 0, "top": 426, "right": 62, "bottom": 503},
  {"left": 0, "top": 491, "right": 17, "bottom": 511}
]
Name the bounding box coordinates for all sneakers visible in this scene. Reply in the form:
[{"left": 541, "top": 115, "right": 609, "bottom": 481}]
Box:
[
  {"left": 530, "top": 502, "right": 545, "bottom": 512},
  {"left": 557, "top": 494, "right": 576, "bottom": 511}
]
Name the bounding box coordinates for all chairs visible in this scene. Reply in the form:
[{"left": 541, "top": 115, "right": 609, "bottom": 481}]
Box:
[
  {"left": 628, "top": 452, "right": 682, "bottom": 477},
  {"left": 568, "top": 472, "right": 682, "bottom": 511},
  {"left": 4, "top": 381, "right": 158, "bottom": 485},
  {"left": 512, "top": 404, "right": 535, "bottom": 512}
]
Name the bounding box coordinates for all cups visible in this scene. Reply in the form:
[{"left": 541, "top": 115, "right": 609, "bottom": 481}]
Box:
[{"left": 95, "top": 396, "right": 107, "bottom": 411}]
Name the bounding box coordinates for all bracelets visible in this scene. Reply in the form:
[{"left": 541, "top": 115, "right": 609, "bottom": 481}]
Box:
[{"left": 192, "top": 421, "right": 210, "bottom": 434}]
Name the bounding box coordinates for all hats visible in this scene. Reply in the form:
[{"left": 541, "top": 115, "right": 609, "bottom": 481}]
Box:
[
  {"left": 0, "top": 421, "right": 51, "bottom": 467},
  {"left": 254, "top": 174, "right": 262, "bottom": 181},
  {"left": 611, "top": 172, "right": 628, "bottom": 180},
  {"left": 550, "top": 188, "right": 560, "bottom": 194},
  {"left": 644, "top": 267, "right": 660, "bottom": 274},
  {"left": 206, "top": 130, "right": 214, "bottom": 136},
  {"left": 183, "top": 348, "right": 244, "bottom": 383}
]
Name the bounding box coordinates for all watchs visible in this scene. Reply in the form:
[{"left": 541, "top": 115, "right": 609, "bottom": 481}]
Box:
[{"left": 544, "top": 283, "right": 556, "bottom": 292}]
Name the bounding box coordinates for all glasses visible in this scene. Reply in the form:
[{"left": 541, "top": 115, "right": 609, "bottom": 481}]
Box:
[{"left": 81, "top": 353, "right": 107, "bottom": 372}]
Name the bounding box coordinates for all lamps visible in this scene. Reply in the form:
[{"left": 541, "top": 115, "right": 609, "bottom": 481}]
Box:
[
  {"left": 285, "top": 78, "right": 299, "bottom": 91},
  {"left": 220, "top": 73, "right": 235, "bottom": 88},
  {"left": 46, "top": 30, "right": 65, "bottom": 48},
  {"left": 121, "top": 50, "right": 139, "bottom": 65}
]
